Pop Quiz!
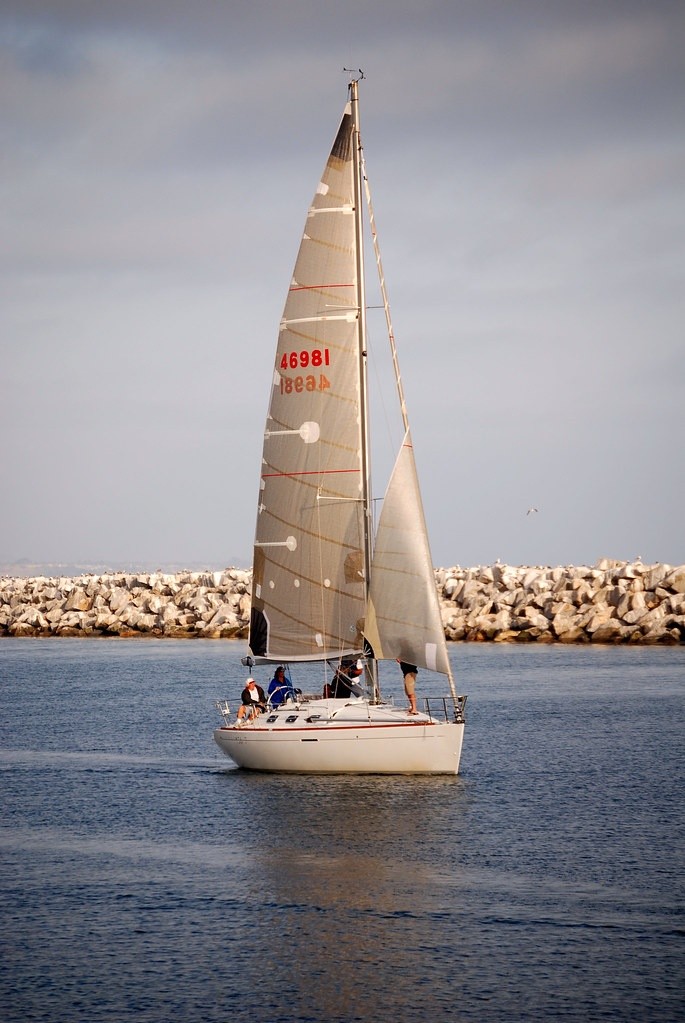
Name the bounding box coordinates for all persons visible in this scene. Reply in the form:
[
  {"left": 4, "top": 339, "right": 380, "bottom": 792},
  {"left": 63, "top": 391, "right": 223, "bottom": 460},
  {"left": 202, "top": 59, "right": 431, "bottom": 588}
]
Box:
[
  {"left": 234, "top": 678, "right": 266, "bottom": 727},
  {"left": 324, "top": 660, "right": 361, "bottom": 699},
  {"left": 396, "top": 638, "right": 418, "bottom": 714},
  {"left": 268, "top": 666, "right": 292, "bottom": 709}
]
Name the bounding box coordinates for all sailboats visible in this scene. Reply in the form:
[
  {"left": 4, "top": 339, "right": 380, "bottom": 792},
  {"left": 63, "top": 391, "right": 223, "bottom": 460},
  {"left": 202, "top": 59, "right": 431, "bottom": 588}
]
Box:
[{"left": 212, "top": 64, "right": 468, "bottom": 775}]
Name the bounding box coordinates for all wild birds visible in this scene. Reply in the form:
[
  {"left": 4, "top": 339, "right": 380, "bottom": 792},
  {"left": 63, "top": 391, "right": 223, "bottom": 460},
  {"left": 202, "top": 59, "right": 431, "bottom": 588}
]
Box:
[
  {"left": 432, "top": 555, "right": 661, "bottom": 574},
  {"left": 527, "top": 508, "right": 539, "bottom": 517},
  {"left": 0, "top": 564, "right": 254, "bottom": 581}
]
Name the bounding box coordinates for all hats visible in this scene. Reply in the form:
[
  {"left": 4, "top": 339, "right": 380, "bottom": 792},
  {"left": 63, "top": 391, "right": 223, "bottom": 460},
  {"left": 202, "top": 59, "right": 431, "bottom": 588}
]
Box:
[{"left": 246, "top": 678, "right": 255, "bottom": 686}]
